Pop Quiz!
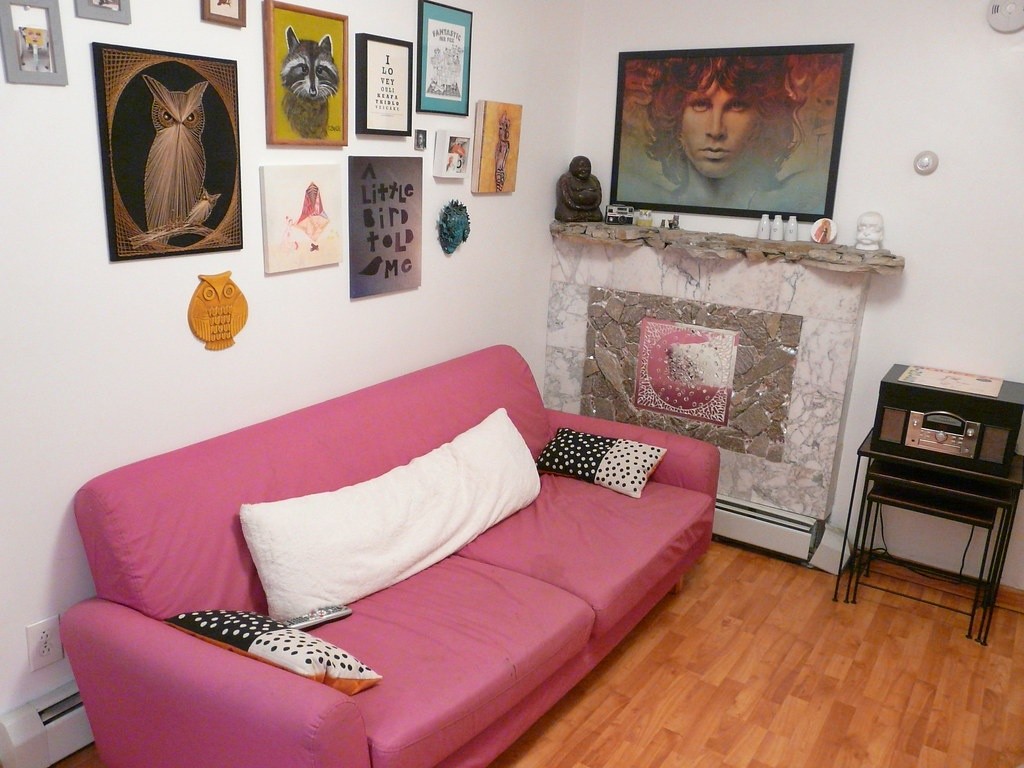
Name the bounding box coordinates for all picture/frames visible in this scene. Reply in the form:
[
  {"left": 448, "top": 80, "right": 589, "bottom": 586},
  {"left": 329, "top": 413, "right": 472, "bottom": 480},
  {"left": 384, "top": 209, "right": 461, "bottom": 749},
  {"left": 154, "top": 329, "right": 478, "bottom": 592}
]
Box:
[
  {"left": 609, "top": 43, "right": 855, "bottom": 222},
  {"left": 72, "top": 0, "right": 132, "bottom": 25},
  {"left": 262, "top": 0, "right": 350, "bottom": 148},
  {"left": 0, "top": 0, "right": 68, "bottom": 84},
  {"left": 416, "top": 0, "right": 473, "bottom": 116},
  {"left": 91, "top": 40, "right": 245, "bottom": 263},
  {"left": 355, "top": 32, "right": 413, "bottom": 138},
  {"left": 199, "top": 0, "right": 247, "bottom": 28}
]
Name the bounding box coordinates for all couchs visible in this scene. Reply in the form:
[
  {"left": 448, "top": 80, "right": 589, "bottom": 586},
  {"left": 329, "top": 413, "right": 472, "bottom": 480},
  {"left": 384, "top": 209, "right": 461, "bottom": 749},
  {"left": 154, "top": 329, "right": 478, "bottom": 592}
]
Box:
[{"left": 56, "top": 345, "right": 725, "bottom": 768}]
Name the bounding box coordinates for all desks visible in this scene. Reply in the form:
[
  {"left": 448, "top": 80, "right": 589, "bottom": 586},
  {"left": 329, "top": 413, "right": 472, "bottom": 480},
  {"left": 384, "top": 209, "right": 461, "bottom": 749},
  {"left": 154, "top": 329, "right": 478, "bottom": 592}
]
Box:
[{"left": 833, "top": 427, "right": 1024, "bottom": 645}]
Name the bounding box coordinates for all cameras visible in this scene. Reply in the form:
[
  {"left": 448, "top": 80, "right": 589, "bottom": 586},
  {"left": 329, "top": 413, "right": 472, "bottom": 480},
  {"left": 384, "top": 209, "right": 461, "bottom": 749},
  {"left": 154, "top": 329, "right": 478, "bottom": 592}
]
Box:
[{"left": 605, "top": 204, "right": 634, "bottom": 225}]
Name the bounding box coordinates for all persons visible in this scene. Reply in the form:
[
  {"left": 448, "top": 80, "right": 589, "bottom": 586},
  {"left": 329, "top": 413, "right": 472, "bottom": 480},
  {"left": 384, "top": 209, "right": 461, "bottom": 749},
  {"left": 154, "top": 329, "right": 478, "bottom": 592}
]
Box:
[
  {"left": 415, "top": 134, "right": 425, "bottom": 150},
  {"left": 856, "top": 212, "right": 885, "bottom": 251},
  {"left": 555, "top": 156, "right": 603, "bottom": 221}
]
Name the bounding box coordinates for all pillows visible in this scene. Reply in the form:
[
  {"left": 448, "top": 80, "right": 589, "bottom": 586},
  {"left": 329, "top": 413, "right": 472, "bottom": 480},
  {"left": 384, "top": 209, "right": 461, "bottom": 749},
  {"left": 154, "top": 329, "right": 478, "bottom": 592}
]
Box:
[
  {"left": 237, "top": 406, "right": 542, "bottom": 618},
  {"left": 535, "top": 427, "right": 668, "bottom": 500},
  {"left": 160, "top": 610, "right": 386, "bottom": 698}
]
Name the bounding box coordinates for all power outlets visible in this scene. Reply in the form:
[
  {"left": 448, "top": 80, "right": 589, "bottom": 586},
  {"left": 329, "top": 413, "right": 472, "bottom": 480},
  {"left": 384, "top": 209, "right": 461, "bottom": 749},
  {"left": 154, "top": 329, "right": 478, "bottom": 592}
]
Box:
[{"left": 26, "top": 615, "right": 66, "bottom": 672}]
